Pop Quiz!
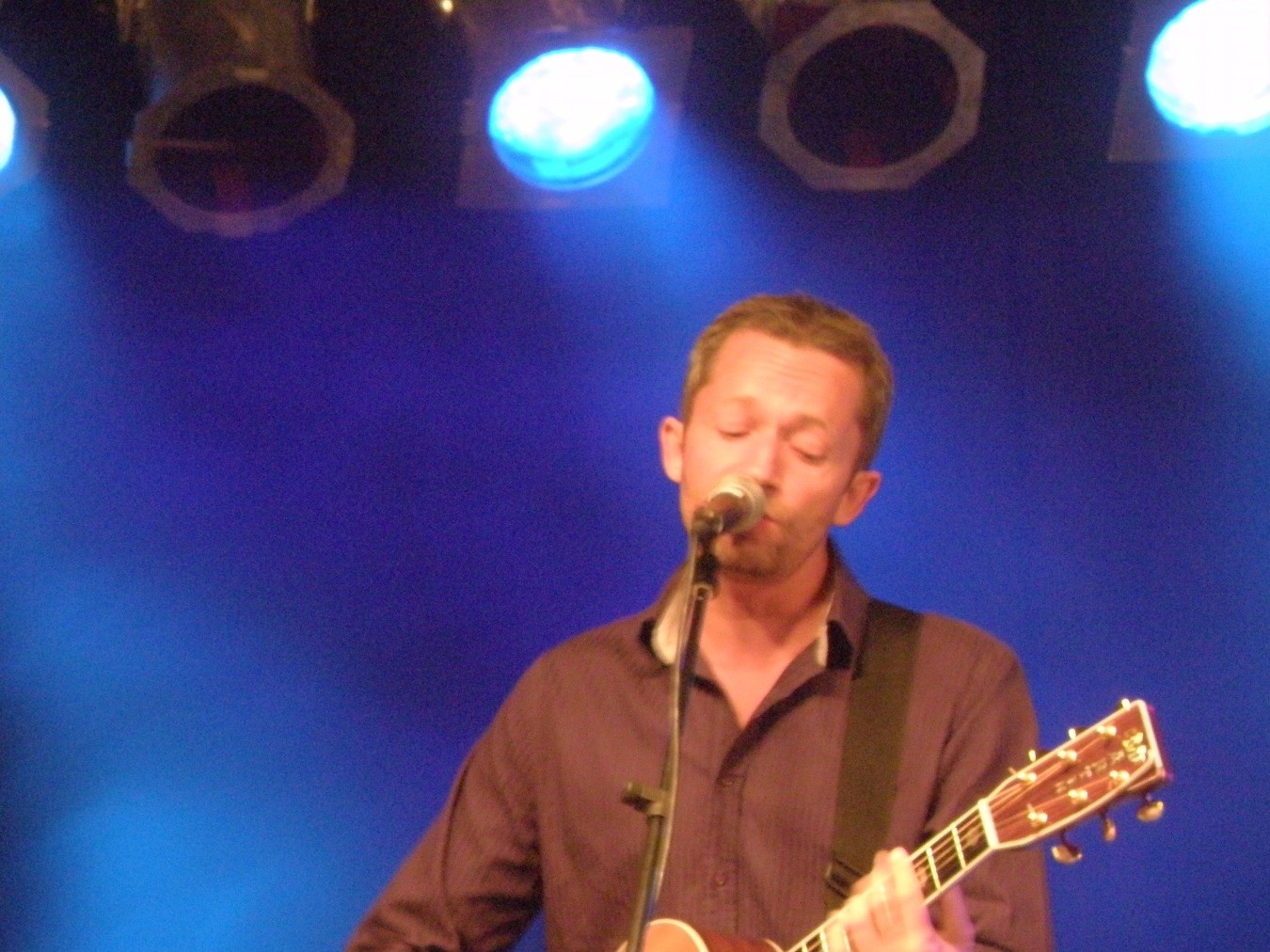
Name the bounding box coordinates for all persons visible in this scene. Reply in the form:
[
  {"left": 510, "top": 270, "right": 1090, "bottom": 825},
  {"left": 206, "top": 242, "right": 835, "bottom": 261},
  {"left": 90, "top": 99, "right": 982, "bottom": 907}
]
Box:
[{"left": 347, "top": 294, "right": 1050, "bottom": 952}]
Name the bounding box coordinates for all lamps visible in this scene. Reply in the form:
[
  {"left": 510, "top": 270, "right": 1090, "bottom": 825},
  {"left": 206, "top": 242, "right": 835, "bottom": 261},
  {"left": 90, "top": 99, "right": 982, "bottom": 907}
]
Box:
[
  {"left": 1109, "top": 0, "right": 1269, "bottom": 164},
  {"left": 451, "top": 0, "right": 696, "bottom": 225},
  {"left": 121, "top": 0, "right": 354, "bottom": 239},
  {"left": 749, "top": 0, "right": 990, "bottom": 191}
]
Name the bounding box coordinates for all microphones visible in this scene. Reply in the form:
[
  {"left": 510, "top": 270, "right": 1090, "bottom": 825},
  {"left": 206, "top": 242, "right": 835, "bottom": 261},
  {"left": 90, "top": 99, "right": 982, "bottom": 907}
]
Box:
[{"left": 694, "top": 476, "right": 767, "bottom": 534}]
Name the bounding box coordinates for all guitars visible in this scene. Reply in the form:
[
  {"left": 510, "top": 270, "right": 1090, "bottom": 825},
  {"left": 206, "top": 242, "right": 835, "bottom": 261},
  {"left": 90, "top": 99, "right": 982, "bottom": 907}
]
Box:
[{"left": 611, "top": 695, "right": 1176, "bottom": 952}]
